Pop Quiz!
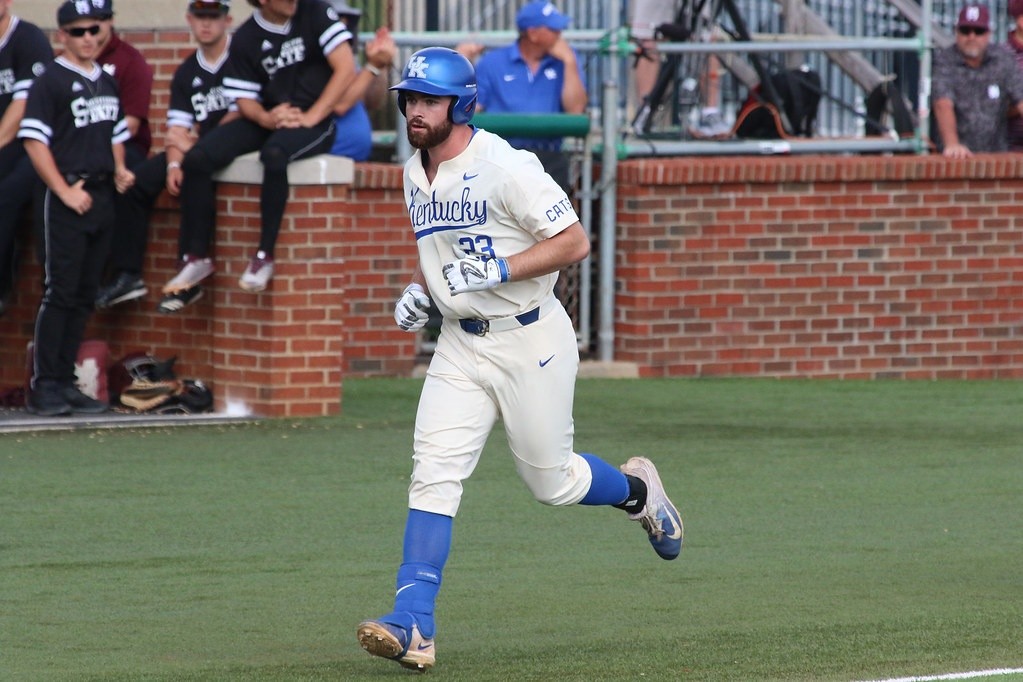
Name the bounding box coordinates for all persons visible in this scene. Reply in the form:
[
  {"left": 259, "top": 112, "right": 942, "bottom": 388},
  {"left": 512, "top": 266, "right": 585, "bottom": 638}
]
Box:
[
  {"left": 331, "top": 0, "right": 395, "bottom": 159},
  {"left": 631, "top": 0, "right": 730, "bottom": 137},
  {"left": 475, "top": 0, "right": 588, "bottom": 151},
  {"left": 0, "top": 0, "right": 152, "bottom": 415},
  {"left": 357, "top": 47, "right": 684, "bottom": 670},
  {"left": 94, "top": 0, "right": 239, "bottom": 309},
  {"left": 162, "top": 0, "right": 356, "bottom": 293},
  {"left": 932, "top": 0, "right": 1023, "bottom": 159}
]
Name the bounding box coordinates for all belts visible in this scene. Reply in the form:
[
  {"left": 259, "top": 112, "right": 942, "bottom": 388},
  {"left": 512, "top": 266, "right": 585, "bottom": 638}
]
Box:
[{"left": 461, "top": 306, "right": 541, "bottom": 336}]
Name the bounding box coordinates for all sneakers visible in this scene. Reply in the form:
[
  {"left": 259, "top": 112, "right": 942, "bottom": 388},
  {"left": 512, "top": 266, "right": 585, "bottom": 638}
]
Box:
[
  {"left": 617, "top": 456, "right": 684, "bottom": 560},
  {"left": 162, "top": 256, "right": 214, "bottom": 296},
  {"left": 58, "top": 387, "right": 107, "bottom": 414},
  {"left": 29, "top": 390, "right": 70, "bottom": 416},
  {"left": 96, "top": 277, "right": 149, "bottom": 309},
  {"left": 357, "top": 620, "right": 435, "bottom": 670},
  {"left": 237, "top": 255, "right": 274, "bottom": 293},
  {"left": 159, "top": 283, "right": 203, "bottom": 313}
]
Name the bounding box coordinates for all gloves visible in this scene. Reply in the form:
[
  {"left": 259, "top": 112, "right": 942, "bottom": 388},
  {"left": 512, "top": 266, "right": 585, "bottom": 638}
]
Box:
[
  {"left": 441, "top": 242, "right": 510, "bottom": 295},
  {"left": 393, "top": 281, "right": 431, "bottom": 331}
]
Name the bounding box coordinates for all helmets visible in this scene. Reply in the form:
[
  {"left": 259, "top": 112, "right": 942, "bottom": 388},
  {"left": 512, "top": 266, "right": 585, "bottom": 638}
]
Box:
[{"left": 387, "top": 45, "right": 478, "bottom": 126}]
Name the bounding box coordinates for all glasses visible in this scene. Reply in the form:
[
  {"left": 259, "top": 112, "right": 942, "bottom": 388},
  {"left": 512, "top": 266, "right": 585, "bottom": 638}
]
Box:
[
  {"left": 961, "top": 26, "right": 988, "bottom": 38},
  {"left": 191, "top": 2, "right": 227, "bottom": 11},
  {"left": 65, "top": 25, "right": 100, "bottom": 37}
]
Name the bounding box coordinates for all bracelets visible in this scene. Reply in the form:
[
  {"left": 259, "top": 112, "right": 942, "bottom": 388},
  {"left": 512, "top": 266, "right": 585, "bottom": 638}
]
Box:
[
  {"left": 168, "top": 162, "right": 180, "bottom": 170},
  {"left": 367, "top": 64, "right": 380, "bottom": 76}
]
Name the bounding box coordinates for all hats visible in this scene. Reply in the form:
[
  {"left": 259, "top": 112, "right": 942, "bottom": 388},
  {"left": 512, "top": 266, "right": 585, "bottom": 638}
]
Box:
[
  {"left": 87, "top": 0, "right": 113, "bottom": 16},
  {"left": 517, "top": 0, "right": 572, "bottom": 32},
  {"left": 188, "top": 0, "right": 230, "bottom": 16},
  {"left": 58, "top": 0, "right": 102, "bottom": 25},
  {"left": 958, "top": 5, "right": 988, "bottom": 28}
]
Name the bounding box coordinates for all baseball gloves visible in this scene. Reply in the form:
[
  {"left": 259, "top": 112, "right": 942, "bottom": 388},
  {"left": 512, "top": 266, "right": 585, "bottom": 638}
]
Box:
[{"left": 121, "top": 381, "right": 179, "bottom": 413}]
